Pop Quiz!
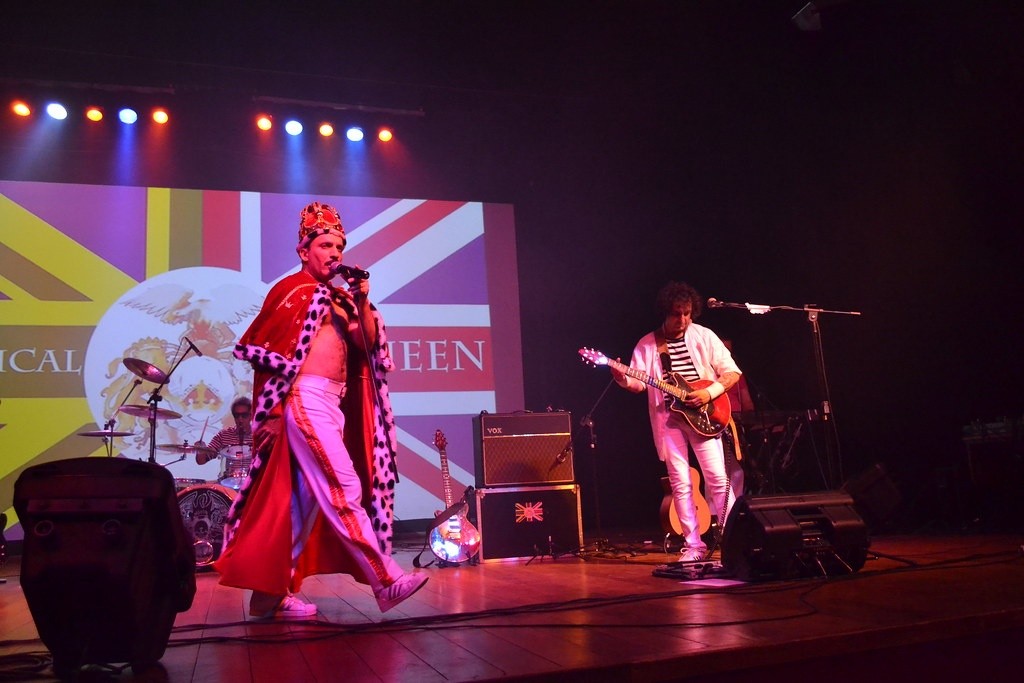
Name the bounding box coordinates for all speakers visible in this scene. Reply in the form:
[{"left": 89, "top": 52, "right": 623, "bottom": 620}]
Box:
[
  {"left": 472, "top": 411, "right": 575, "bottom": 488},
  {"left": 721, "top": 492, "right": 867, "bottom": 582},
  {"left": 13, "top": 455, "right": 196, "bottom": 672},
  {"left": 967, "top": 436, "right": 1024, "bottom": 533}
]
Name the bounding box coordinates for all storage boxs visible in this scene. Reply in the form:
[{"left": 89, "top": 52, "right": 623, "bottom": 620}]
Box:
[{"left": 465, "top": 483, "right": 585, "bottom": 563}]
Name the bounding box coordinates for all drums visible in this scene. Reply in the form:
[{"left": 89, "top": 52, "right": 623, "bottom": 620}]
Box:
[
  {"left": 174, "top": 478, "right": 206, "bottom": 492},
  {"left": 217, "top": 442, "right": 252, "bottom": 490},
  {"left": 175, "top": 482, "right": 238, "bottom": 568}
]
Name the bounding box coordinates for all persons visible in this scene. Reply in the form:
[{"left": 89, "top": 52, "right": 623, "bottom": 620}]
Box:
[
  {"left": 194, "top": 396, "right": 251, "bottom": 481},
  {"left": 716, "top": 331, "right": 754, "bottom": 497},
  {"left": 610, "top": 282, "right": 742, "bottom": 566},
  {"left": 212, "top": 199, "right": 430, "bottom": 617}
]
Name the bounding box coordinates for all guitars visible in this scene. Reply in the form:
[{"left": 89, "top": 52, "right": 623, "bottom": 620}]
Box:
[
  {"left": 427, "top": 428, "right": 482, "bottom": 564},
  {"left": 578, "top": 346, "right": 733, "bottom": 439},
  {"left": 660, "top": 464, "right": 712, "bottom": 540}
]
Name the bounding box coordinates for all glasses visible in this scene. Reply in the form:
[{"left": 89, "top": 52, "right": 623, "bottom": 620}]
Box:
[{"left": 233, "top": 411, "right": 249, "bottom": 418}]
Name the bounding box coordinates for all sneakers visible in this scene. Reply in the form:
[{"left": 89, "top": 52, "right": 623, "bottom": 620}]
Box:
[
  {"left": 249, "top": 595, "right": 317, "bottom": 617},
  {"left": 678, "top": 547, "right": 704, "bottom": 562},
  {"left": 376, "top": 571, "right": 430, "bottom": 613}
]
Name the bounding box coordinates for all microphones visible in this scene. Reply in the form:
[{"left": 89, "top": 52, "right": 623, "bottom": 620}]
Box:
[
  {"left": 557, "top": 448, "right": 569, "bottom": 462},
  {"left": 185, "top": 337, "right": 202, "bottom": 357},
  {"left": 708, "top": 298, "right": 724, "bottom": 308},
  {"left": 330, "top": 262, "right": 370, "bottom": 279}
]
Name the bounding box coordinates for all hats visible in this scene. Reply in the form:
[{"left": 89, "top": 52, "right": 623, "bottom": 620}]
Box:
[{"left": 295, "top": 203, "right": 348, "bottom": 252}]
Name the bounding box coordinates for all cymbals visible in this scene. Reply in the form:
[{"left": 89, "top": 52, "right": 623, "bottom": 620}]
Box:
[
  {"left": 117, "top": 404, "right": 183, "bottom": 420},
  {"left": 154, "top": 443, "right": 219, "bottom": 456},
  {"left": 75, "top": 430, "right": 135, "bottom": 438},
  {"left": 122, "top": 356, "right": 170, "bottom": 385}
]
